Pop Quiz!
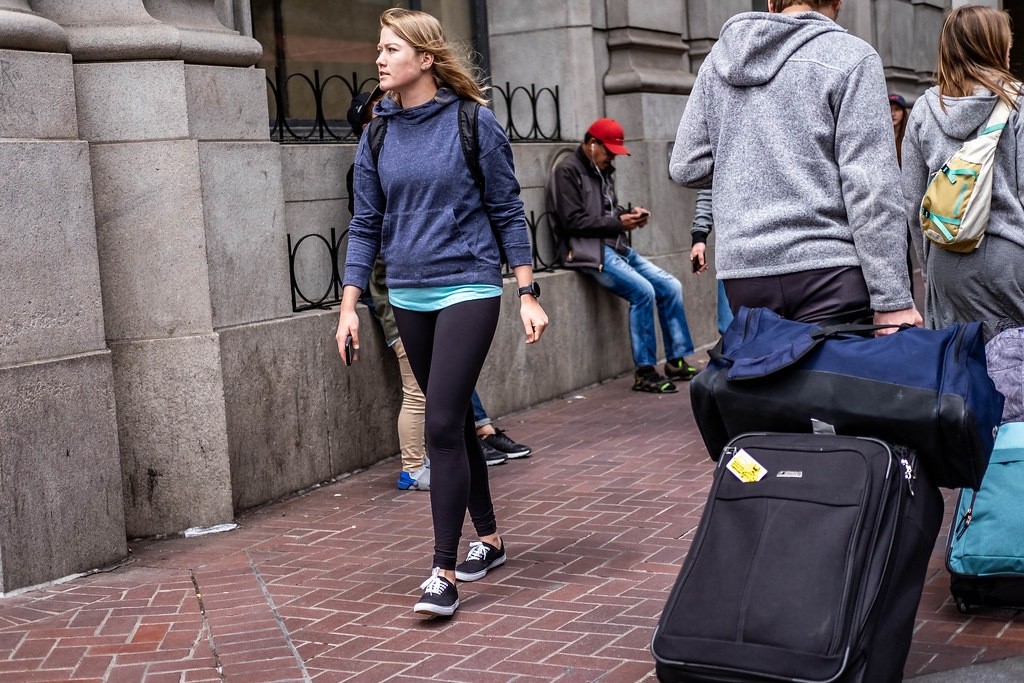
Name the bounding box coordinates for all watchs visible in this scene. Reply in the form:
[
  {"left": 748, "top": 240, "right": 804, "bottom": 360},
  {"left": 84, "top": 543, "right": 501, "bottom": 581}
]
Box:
[{"left": 517, "top": 281, "right": 540, "bottom": 298}]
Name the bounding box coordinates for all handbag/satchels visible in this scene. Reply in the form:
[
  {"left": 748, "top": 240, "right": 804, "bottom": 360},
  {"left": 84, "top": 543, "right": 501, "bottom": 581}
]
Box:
[
  {"left": 688, "top": 304, "right": 1005, "bottom": 493},
  {"left": 919, "top": 81, "right": 1020, "bottom": 253}
]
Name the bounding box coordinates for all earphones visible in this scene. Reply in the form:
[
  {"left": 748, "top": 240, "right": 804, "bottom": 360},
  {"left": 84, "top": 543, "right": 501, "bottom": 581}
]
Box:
[{"left": 591, "top": 143, "right": 595, "bottom": 150}]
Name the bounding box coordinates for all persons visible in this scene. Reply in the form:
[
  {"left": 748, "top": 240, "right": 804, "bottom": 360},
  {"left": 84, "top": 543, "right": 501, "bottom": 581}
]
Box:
[
  {"left": 471, "top": 390, "right": 532, "bottom": 466},
  {"left": 901, "top": 6, "right": 1024, "bottom": 334},
  {"left": 548, "top": 119, "right": 702, "bottom": 392},
  {"left": 346, "top": 95, "right": 430, "bottom": 489},
  {"left": 689, "top": 189, "right": 734, "bottom": 334},
  {"left": 335, "top": 9, "right": 548, "bottom": 614},
  {"left": 888, "top": 93, "right": 915, "bottom": 300},
  {"left": 668, "top": 0, "right": 922, "bottom": 337}
]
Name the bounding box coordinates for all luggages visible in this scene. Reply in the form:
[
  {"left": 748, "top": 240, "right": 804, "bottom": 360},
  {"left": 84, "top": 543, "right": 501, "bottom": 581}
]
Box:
[
  {"left": 945, "top": 422, "right": 1024, "bottom": 616},
  {"left": 651, "top": 429, "right": 944, "bottom": 683}
]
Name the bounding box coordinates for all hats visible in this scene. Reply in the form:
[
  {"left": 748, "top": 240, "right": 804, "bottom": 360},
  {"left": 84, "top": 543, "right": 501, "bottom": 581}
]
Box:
[
  {"left": 588, "top": 118, "right": 632, "bottom": 157},
  {"left": 347, "top": 83, "right": 379, "bottom": 129},
  {"left": 887, "top": 94, "right": 906, "bottom": 106}
]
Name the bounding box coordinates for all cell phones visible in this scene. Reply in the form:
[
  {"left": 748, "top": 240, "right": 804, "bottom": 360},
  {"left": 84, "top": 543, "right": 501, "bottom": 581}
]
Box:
[
  {"left": 638, "top": 213, "right": 649, "bottom": 218},
  {"left": 346, "top": 335, "right": 354, "bottom": 366},
  {"left": 691, "top": 253, "right": 706, "bottom": 273}
]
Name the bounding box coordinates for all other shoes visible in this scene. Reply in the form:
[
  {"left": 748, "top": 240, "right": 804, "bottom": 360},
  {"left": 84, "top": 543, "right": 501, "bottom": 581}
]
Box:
[
  {"left": 631, "top": 370, "right": 678, "bottom": 394},
  {"left": 664, "top": 358, "right": 702, "bottom": 381}
]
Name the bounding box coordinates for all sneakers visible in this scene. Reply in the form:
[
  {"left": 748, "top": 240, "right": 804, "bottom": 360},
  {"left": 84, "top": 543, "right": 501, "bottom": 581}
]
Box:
[
  {"left": 414, "top": 566, "right": 459, "bottom": 616},
  {"left": 480, "top": 427, "right": 532, "bottom": 459},
  {"left": 477, "top": 436, "right": 508, "bottom": 466},
  {"left": 396, "top": 469, "right": 430, "bottom": 491},
  {"left": 455, "top": 537, "right": 507, "bottom": 582}
]
